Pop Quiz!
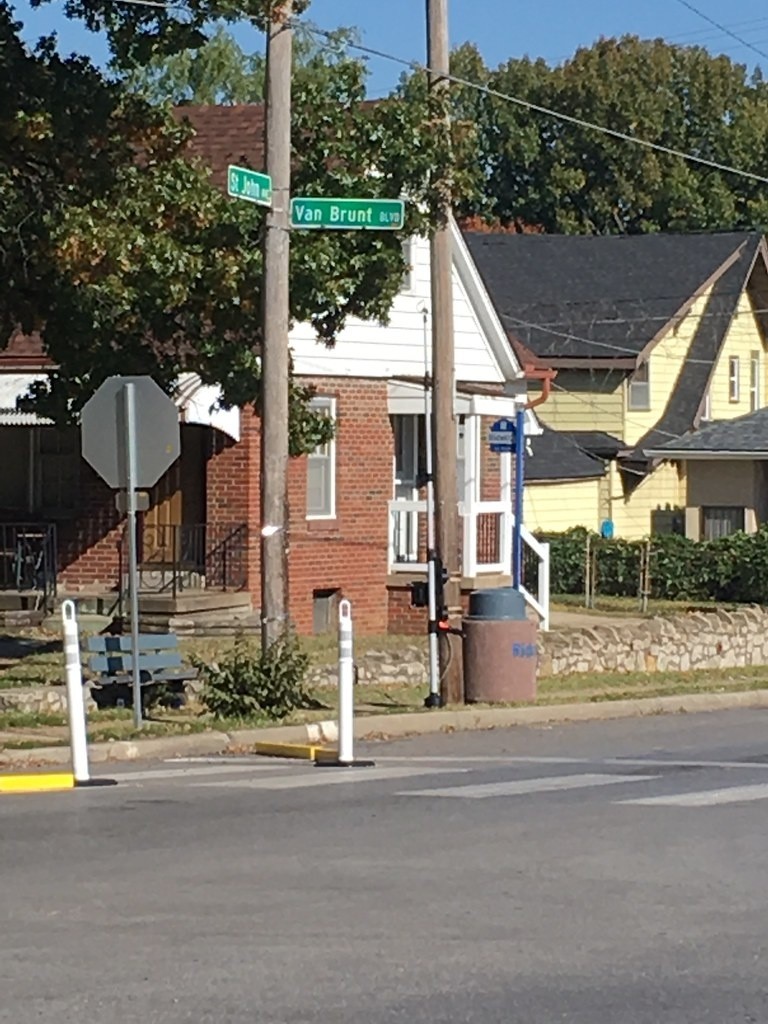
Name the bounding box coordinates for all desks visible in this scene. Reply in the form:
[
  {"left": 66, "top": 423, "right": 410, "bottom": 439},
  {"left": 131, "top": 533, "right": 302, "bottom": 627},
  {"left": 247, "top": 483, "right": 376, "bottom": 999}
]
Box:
[{"left": 0, "top": 532, "right": 48, "bottom": 590}]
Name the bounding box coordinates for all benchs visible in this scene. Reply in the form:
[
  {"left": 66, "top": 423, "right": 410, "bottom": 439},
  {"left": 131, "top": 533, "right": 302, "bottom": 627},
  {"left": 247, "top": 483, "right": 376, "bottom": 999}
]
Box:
[{"left": 86, "top": 635, "right": 198, "bottom": 707}]
